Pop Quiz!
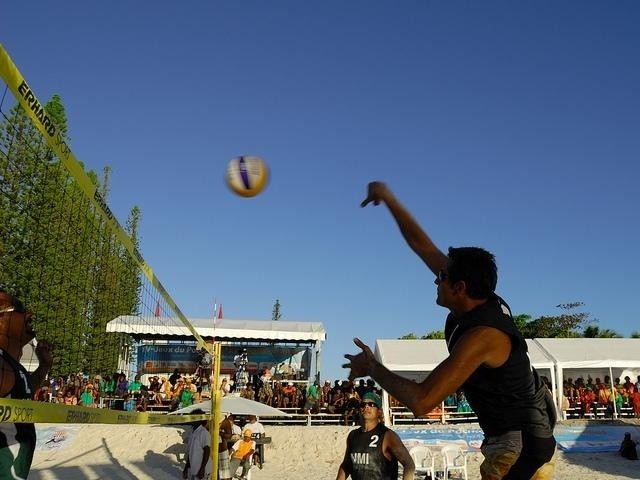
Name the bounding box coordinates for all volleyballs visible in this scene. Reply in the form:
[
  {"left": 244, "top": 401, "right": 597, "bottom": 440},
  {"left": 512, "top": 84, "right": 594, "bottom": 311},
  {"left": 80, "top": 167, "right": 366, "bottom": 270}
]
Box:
[{"left": 226, "top": 158, "right": 267, "bottom": 196}]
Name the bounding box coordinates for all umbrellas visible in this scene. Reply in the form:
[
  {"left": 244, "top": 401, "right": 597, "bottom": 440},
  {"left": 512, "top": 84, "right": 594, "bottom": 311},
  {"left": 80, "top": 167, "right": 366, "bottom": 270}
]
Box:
[{"left": 167, "top": 393, "right": 292, "bottom": 416}]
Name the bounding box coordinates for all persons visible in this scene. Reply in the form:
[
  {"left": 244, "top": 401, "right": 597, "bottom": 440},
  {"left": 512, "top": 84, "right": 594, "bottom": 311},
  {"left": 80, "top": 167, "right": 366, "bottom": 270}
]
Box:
[
  {"left": 540, "top": 374, "right": 640, "bottom": 418},
  {"left": 228, "top": 415, "right": 242, "bottom": 435},
  {"left": 342, "top": 181, "right": 557, "bottom": 480},
  {"left": 242, "top": 415, "right": 265, "bottom": 465},
  {"left": 206, "top": 415, "right": 232, "bottom": 480},
  {"left": 336, "top": 392, "right": 415, "bottom": 480},
  {"left": 0, "top": 289, "right": 56, "bottom": 480},
  {"left": 182, "top": 408, "right": 212, "bottom": 480},
  {"left": 619, "top": 432, "right": 637, "bottom": 460},
  {"left": 228, "top": 429, "right": 256, "bottom": 480}
]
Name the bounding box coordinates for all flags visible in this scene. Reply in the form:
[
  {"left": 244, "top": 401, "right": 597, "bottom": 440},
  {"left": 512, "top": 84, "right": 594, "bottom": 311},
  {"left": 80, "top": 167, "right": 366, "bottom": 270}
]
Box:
[
  {"left": 218, "top": 304, "right": 222, "bottom": 319},
  {"left": 155, "top": 301, "right": 160, "bottom": 316}
]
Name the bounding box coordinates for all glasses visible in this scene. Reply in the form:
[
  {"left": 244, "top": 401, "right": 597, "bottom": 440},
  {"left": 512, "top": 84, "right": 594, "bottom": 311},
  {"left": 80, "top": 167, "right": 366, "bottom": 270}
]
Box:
[
  {"left": 0, "top": 301, "right": 27, "bottom": 315},
  {"left": 361, "top": 401, "right": 377, "bottom": 409}
]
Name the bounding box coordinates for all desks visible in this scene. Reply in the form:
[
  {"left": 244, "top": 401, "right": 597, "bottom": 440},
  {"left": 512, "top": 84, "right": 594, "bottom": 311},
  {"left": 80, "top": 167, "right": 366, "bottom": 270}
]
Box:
[{"left": 227, "top": 437, "right": 272, "bottom": 469}]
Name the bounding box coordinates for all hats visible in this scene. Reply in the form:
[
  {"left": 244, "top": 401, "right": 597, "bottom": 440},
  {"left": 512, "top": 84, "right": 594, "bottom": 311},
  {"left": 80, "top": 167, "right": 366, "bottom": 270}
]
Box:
[{"left": 243, "top": 429, "right": 252, "bottom": 437}]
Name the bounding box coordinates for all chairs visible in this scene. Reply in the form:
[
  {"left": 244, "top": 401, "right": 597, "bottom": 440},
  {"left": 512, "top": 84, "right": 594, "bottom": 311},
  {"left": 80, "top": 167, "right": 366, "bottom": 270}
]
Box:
[
  {"left": 441, "top": 445, "right": 468, "bottom": 480},
  {"left": 409, "top": 446, "right": 435, "bottom": 480}
]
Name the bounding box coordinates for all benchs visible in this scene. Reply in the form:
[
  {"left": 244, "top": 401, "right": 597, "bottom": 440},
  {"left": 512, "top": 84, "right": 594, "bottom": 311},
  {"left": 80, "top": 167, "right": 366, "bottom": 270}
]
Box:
[{"left": 41, "top": 379, "right": 640, "bottom": 421}]
